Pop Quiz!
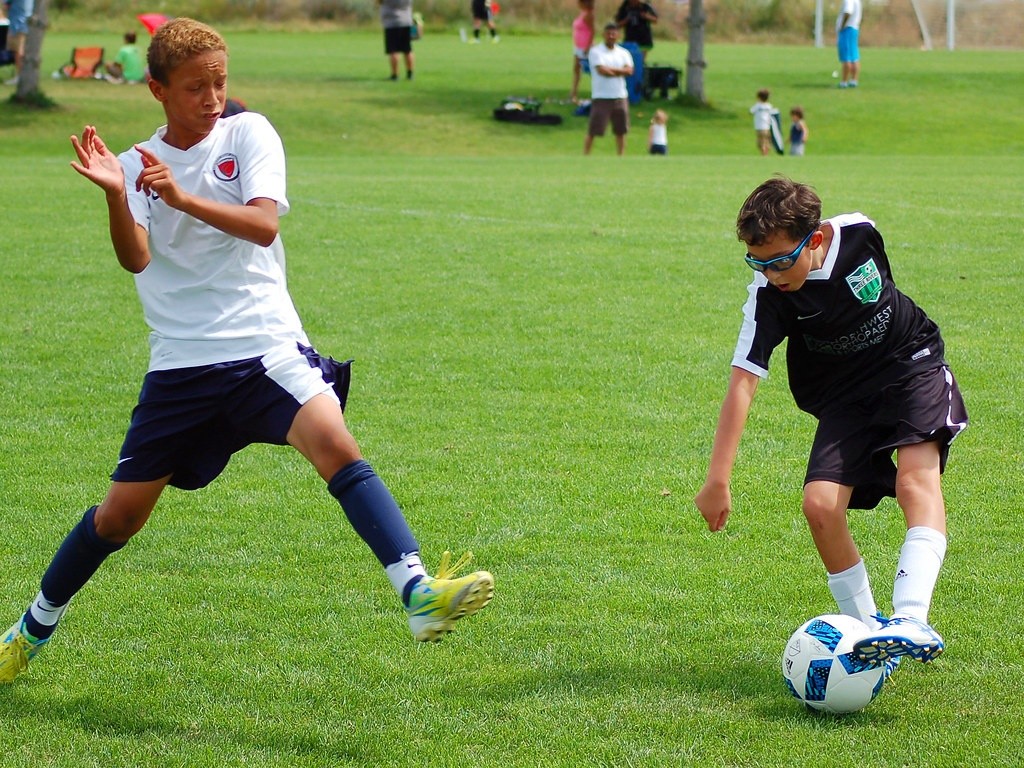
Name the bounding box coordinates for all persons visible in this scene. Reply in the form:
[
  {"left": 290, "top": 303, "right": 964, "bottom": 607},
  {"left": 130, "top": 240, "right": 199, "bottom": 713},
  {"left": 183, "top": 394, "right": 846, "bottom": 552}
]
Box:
[
  {"left": 614, "top": 0, "right": 659, "bottom": 61},
  {"left": 3, "top": 0, "right": 34, "bottom": 84},
  {"left": 0, "top": 20, "right": 494, "bottom": 685},
  {"left": 693, "top": 173, "right": 968, "bottom": 667},
  {"left": 377, "top": 0, "right": 416, "bottom": 81},
  {"left": 648, "top": 110, "right": 669, "bottom": 155},
  {"left": 567, "top": 0, "right": 596, "bottom": 101},
  {"left": 784, "top": 106, "right": 809, "bottom": 155},
  {"left": 835, "top": 0, "right": 863, "bottom": 89},
  {"left": 582, "top": 25, "right": 634, "bottom": 156},
  {"left": 470, "top": 0, "right": 499, "bottom": 44},
  {"left": 105, "top": 30, "right": 144, "bottom": 81},
  {"left": 749, "top": 89, "right": 774, "bottom": 156}
]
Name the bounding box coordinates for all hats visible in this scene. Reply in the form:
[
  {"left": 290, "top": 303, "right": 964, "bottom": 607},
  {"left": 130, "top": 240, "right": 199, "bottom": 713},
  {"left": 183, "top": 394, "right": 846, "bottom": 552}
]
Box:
[{"left": 604, "top": 23, "right": 617, "bottom": 29}]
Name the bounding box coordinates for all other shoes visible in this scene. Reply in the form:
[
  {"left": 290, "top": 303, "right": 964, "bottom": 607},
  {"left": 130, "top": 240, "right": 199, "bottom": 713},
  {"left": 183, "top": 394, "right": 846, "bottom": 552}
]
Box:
[
  {"left": 490, "top": 36, "right": 500, "bottom": 43},
  {"left": 837, "top": 83, "right": 848, "bottom": 88},
  {"left": 848, "top": 81, "right": 857, "bottom": 87},
  {"left": 467, "top": 38, "right": 480, "bottom": 44}
]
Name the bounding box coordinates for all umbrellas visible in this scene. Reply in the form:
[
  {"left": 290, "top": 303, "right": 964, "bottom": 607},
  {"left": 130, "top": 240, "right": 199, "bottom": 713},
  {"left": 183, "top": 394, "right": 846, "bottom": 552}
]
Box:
[{"left": 137, "top": 14, "right": 170, "bottom": 33}]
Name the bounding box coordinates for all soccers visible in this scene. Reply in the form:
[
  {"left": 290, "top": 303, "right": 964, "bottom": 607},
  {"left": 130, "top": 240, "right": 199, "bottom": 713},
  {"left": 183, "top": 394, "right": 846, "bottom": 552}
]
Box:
[{"left": 780, "top": 614, "right": 888, "bottom": 716}]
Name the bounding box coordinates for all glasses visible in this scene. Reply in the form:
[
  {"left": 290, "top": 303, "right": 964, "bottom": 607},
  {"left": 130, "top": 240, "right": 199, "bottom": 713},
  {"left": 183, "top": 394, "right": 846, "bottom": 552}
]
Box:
[{"left": 744, "top": 229, "right": 816, "bottom": 272}]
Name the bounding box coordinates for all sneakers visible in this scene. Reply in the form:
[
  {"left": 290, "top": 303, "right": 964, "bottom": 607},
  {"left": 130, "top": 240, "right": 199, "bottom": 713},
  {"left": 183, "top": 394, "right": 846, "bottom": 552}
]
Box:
[
  {"left": 402, "top": 550, "right": 495, "bottom": 642},
  {"left": 853, "top": 612, "right": 944, "bottom": 666},
  {"left": 0, "top": 611, "right": 55, "bottom": 682}
]
